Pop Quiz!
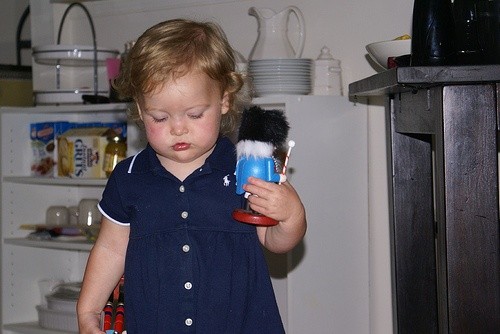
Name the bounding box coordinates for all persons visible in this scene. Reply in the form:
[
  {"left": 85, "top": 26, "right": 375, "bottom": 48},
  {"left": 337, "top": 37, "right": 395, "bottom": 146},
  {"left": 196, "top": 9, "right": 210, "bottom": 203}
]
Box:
[{"left": 77, "top": 19, "right": 307, "bottom": 334}]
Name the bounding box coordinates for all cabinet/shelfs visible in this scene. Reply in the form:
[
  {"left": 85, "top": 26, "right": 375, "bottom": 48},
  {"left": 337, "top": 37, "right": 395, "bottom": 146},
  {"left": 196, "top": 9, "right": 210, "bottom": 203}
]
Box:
[{"left": 0, "top": 95, "right": 372, "bottom": 334}]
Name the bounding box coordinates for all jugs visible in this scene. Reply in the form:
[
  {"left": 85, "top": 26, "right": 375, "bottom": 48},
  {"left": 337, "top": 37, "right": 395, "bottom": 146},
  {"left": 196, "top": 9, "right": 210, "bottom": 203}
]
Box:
[{"left": 248, "top": 6, "right": 305, "bottom": 60}]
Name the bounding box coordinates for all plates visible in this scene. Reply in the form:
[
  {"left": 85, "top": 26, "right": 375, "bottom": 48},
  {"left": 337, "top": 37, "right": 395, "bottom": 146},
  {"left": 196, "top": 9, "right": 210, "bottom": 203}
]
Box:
[{"left": 249, "top": 59, "right": 311, "bottom": 96}]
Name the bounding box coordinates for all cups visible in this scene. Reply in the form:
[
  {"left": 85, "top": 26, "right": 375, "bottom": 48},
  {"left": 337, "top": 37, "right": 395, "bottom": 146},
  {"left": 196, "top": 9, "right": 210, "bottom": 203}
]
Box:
[
  {"left": 47, "top": 206, "right": 70, "bottom": 228},
  {"left": 78, "top": 199, "right": 102, "bottom": 225},
  {"left": 69, "top": 206, "right": 80, "bottom": 225}
]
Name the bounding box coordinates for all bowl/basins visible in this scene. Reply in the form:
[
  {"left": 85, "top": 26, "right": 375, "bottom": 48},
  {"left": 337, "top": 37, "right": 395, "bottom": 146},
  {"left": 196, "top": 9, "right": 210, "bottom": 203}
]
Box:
[{"left": 366, "top": 40, "right": 411, "bottom": 70}]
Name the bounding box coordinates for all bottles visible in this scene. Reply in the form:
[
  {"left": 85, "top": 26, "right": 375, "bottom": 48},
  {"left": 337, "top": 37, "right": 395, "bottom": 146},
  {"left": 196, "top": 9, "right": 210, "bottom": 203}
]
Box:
[
  {"left": 104, "top": 134, "right": 126, "bottom": 177},
  {"left": 313, "top": 47, "right": 342, "bottom": 97}
]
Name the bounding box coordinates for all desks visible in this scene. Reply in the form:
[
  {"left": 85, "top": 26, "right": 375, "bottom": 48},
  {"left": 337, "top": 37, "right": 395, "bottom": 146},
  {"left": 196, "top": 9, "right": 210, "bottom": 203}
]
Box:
[{"left": 349, "top": 65, "right": 500, "bottom": 334}]
